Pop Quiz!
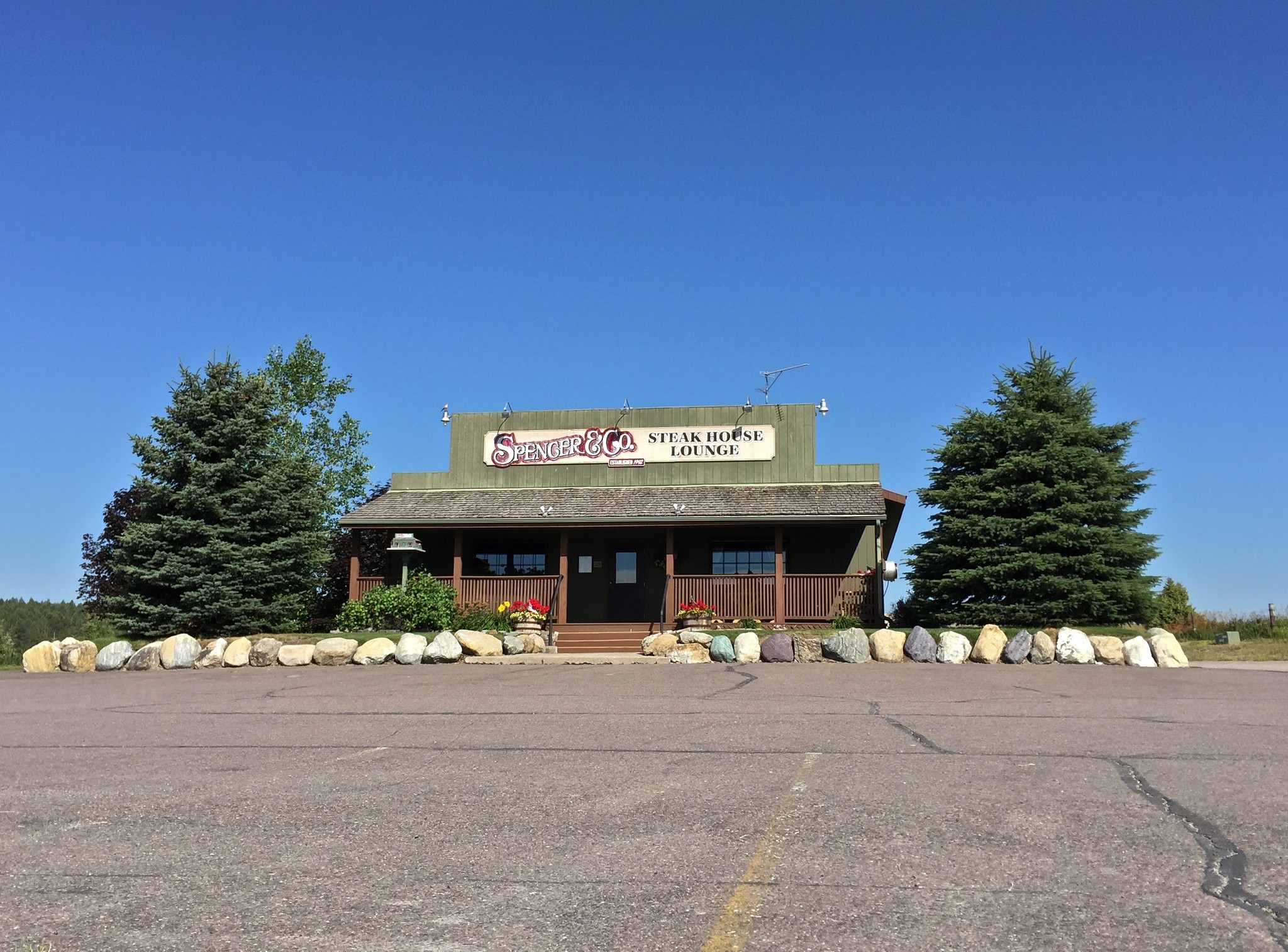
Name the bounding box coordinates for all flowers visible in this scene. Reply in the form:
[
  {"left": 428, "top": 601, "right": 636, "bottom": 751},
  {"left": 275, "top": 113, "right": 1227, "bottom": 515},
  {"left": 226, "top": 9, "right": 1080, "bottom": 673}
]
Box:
[
  {"left": 677, "top": 600, "right": 716, "bottom": 619},
  {"left": 498, "top": 599, "right": 550, "bottom": 620}
]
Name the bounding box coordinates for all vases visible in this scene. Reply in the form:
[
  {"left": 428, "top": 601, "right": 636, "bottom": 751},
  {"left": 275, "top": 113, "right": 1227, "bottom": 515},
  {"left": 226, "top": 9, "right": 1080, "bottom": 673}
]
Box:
[
  {"left": 683, "top": 613, "right": 711, "bottom": 630},
  {"left": 514, "top": 616, "right": 543, "bottom": 632}
]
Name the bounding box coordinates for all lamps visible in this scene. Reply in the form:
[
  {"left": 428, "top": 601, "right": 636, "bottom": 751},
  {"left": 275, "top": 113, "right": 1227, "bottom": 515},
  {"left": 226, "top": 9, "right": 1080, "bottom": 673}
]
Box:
[
  {"left": 539, "top": 505, "right": 553, "bottom": 517},
  {"left": 673, "top": 503, "right": 685, "bottom": 516},
  {"left": 620, "top": 399, "right": 631, "bottom": 414},
  {"left": 815, "top": 398, "right": 829, "bottom": 416},
  {"left": 742, "top": 396, "right": 752, "bottom": 413},
  {"left": 440, "top": 403, "right": 451, "bottom": 426},
  {"left": 501, "top": 402, "right": 513, "bottom": 418}
]
